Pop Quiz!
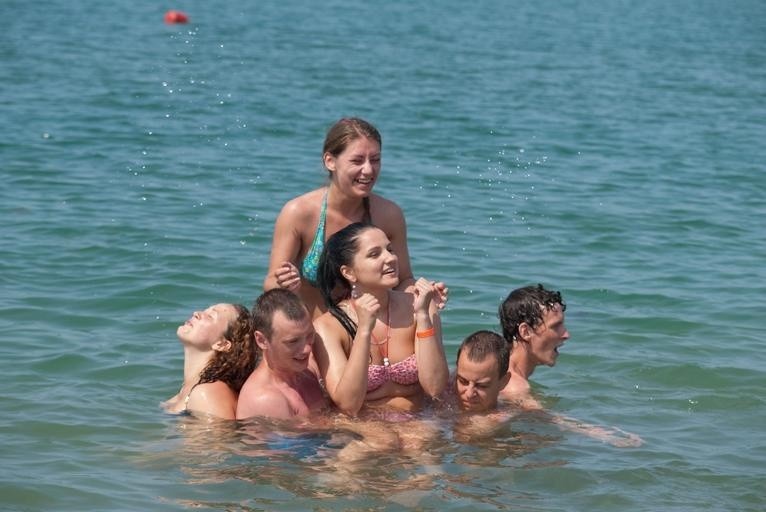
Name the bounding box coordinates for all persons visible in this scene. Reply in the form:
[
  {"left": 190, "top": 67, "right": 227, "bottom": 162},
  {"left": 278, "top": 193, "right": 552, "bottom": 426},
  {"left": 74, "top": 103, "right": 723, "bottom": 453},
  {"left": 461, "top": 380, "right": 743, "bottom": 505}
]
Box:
[
  {"left": 435, "top": 331, "right": 522, "bottom": 445},
  {"left": 312, "top": 224, "right": 449, "bottom": 498},
  {"left": 160, "top": 302, "right": 256, "bottom": 421},
  {"left": 500, "top": 283, "right": 643, "bottom": 449},
  {"left": 264, "top": 117, "right": 448, "bottom": 390},
  {"left": 238, "top": 288, "right": 326, "bottom": 426}
]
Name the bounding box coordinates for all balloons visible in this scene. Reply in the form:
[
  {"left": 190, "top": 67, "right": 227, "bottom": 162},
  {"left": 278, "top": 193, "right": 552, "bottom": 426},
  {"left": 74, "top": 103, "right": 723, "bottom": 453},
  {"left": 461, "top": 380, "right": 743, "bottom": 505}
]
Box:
[{"left": 167, "top": 11, "right": 187, "bottom": 24}]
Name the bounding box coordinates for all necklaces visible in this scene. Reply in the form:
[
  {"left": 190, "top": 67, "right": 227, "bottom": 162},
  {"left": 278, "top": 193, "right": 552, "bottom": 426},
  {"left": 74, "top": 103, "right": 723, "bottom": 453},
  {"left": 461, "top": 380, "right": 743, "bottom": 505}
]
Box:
[
  {"left": 346, "top": 299, "right": 391, "bottom": 345},
  {"left": 351, "top": 296, "right": 396, "bottom": 367}
]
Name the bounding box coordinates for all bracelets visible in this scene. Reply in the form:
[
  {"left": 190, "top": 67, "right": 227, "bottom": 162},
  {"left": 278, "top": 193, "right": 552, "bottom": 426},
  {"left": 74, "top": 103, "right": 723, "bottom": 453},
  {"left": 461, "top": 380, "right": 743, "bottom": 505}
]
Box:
[{"left": 413, "top": 327, "right": 436, "bottom": 339}]
